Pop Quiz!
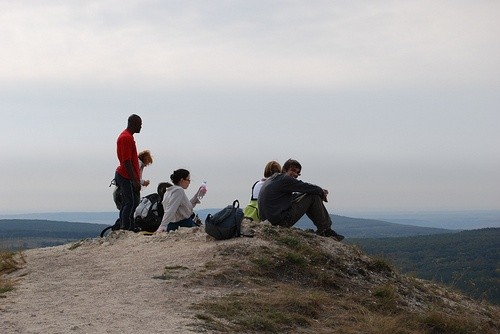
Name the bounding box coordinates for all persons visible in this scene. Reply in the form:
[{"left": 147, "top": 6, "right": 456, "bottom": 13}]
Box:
[
  {"left": 153, "top": 169, "right": 207, "bottom": 236},
  {"left": 251, "top": 160, "right": 282, "bottom": 222},
  {"left": 113, "top": 114, "right": 153, "bottom": 232},
  {"left": 143, "top": 182, "right": 174, "bottom": 232},
  {"left": 257, "top": 158, "right": 344, "bottom": 241}
]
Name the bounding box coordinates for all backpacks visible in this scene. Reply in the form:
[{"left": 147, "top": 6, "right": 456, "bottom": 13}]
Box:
[
  {"left": 205, "top": 200, "right": 244, "bottom": 240},
  {"left": 132, "top": 193, "right": 163, "bottom": 232},
  {"left": 241, "top": 180, "right": 261, "bottom": 223}
]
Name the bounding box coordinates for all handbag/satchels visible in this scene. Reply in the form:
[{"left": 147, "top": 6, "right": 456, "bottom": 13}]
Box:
[{"left": 167, "top": 217, "right": 198, "bottom": 232}]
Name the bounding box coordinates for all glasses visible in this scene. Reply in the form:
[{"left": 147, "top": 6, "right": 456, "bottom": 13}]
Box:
[
  {"left": 288, "top": 168, "right": 301, "bottom": 176},
  {"left": 184, "top": 179, "right": 191, "bottom": 183}
]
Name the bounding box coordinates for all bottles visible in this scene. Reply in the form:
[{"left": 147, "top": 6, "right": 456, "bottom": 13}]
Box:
[{"left": 197, "top": 182, "right": 207, "bottom": 200}]
